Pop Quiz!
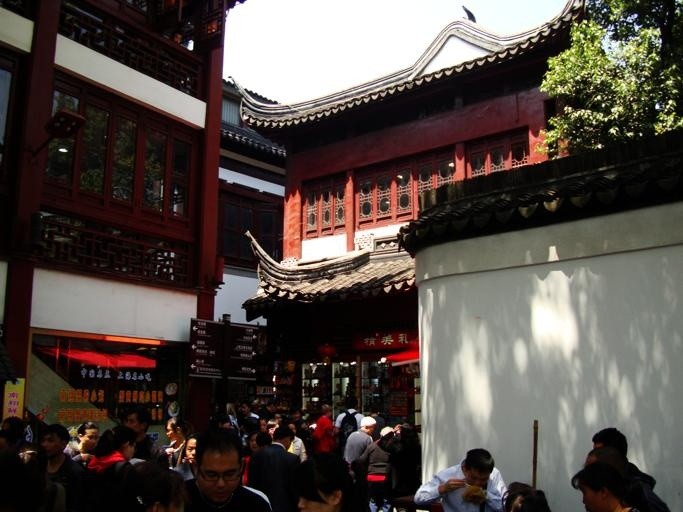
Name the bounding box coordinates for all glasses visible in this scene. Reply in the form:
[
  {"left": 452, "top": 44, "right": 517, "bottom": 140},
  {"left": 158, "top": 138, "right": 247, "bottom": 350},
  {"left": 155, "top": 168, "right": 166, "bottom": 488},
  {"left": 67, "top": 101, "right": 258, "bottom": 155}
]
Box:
[{"left": 199, "top": 467, "right": 238, "bottom": 477}]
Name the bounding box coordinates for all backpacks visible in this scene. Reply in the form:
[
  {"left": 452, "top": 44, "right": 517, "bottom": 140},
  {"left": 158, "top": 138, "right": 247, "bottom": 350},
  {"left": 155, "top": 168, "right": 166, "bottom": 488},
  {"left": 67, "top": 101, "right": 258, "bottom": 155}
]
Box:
[{"left": 340, "top": 411, "right": 359, "bottom": 438}]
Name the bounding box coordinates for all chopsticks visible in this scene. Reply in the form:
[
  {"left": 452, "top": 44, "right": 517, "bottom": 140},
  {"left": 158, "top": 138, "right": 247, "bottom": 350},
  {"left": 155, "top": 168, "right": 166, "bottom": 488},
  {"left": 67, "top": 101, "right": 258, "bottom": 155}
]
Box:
[{"left": 462, "top": 482, "right": 471, "bottom": 488}]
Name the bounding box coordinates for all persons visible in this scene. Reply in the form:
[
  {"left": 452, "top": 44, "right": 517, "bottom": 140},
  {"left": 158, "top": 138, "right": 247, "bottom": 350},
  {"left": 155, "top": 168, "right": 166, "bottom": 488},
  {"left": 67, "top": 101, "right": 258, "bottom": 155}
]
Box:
[
  {"left": 1, "top": 381, "right": 421, "bottom": 512},
  {"left": 583, "top": 449, "right": 630, "bottom": 473},
  {"left": 498, "top": 482, "right": 553, "bottom": 511},
  {"left": 569, "top": 466, "right": 641, "bottom": 511},
  {"left": 590, "top": 426, "right": 674, "bottom": 510},
  {"left": 413, "top": 445, "right": 509, "bottom": 512}
]
